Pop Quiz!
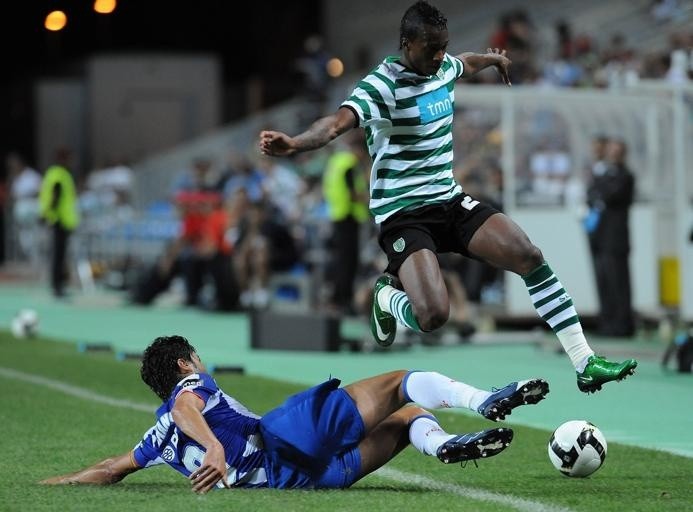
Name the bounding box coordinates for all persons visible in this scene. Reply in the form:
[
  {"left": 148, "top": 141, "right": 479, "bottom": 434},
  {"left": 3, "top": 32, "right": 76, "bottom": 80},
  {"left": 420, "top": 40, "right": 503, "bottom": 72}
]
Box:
[
  {"left": 259, "top": 2, "right": 638, "bottom": 393},
  {"left": 39, "top": 336, "right": 551, "bottom": 490},
  {"left": 392, "top": 104, "right": 607, "bottom": 348},
  {"left": 295, "top": 36, "right": 327, "bottom": 132},
  {"left": 128, "top": 128, "right": 389, "bottom": 318},
  {"left": 579, "top": 127, "right": 637, "bottom": 336},
  {"left": 454, "top": 1, "right": 692, "bottom": 89},
  {"left": 1, "top": 143, "right": 138, "bottom": 296}
]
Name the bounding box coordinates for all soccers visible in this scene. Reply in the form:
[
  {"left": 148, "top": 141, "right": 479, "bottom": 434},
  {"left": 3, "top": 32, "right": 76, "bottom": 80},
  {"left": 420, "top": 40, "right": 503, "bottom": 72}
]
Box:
[{"left": 547, "top": 420, "right": 608, "bottom": 477}]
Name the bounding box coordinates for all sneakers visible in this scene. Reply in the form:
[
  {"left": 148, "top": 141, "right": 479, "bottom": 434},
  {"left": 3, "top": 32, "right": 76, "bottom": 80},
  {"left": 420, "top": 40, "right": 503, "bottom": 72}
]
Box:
[
  {"left": 477, "top": 380, "right": 549, "bottom": 421},
  {"left": 576, "top": 355, "right": 637, "bottom": 392},
  {"left": 436, "top": 427, "right": 513, "bottom": 464},
  {"left": 371, "top": 276, "right": 397, "bottom": 347}
]
